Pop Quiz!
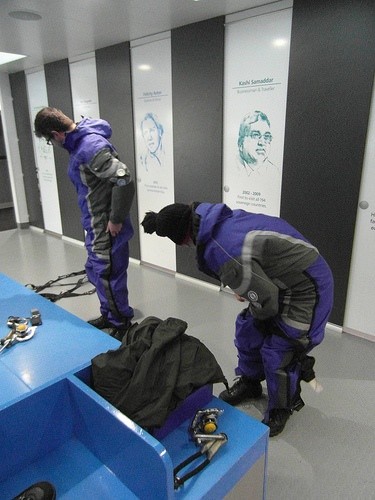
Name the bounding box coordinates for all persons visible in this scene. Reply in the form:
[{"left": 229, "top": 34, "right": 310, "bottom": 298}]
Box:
[
  {"left": 34, "top": 107, "right": 134, "bottom": 342},
  {"left": 142, "top": 202, "right": 334, "bottom": 437}
]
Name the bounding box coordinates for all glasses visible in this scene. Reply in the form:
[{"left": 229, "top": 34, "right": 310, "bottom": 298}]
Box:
[{"left": 47, "top": 135, "right": 53, "bottom": 146}]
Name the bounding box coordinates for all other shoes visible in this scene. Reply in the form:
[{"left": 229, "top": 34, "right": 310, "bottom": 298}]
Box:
[
  {"left": 110, "top": 321, "right": 132, "bottom": 340},
  {"left": 12, "top": 481, "right": 57, "bottom": 500},
  {"left": 88, "top": 316, "right": 108, "bottom": 330}
]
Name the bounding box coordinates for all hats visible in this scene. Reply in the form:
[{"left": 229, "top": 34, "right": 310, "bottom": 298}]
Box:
[{"left": 141, "top": 203, "right": 194, "bottom": 247}]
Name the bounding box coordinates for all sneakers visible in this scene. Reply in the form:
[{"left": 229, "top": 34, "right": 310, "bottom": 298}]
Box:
[
  {"left": 220, "top": 376, "right": 263, "bottom": 405},
  {"left": 261, "top": 407, "right": 292, "bottom": 436}
]
